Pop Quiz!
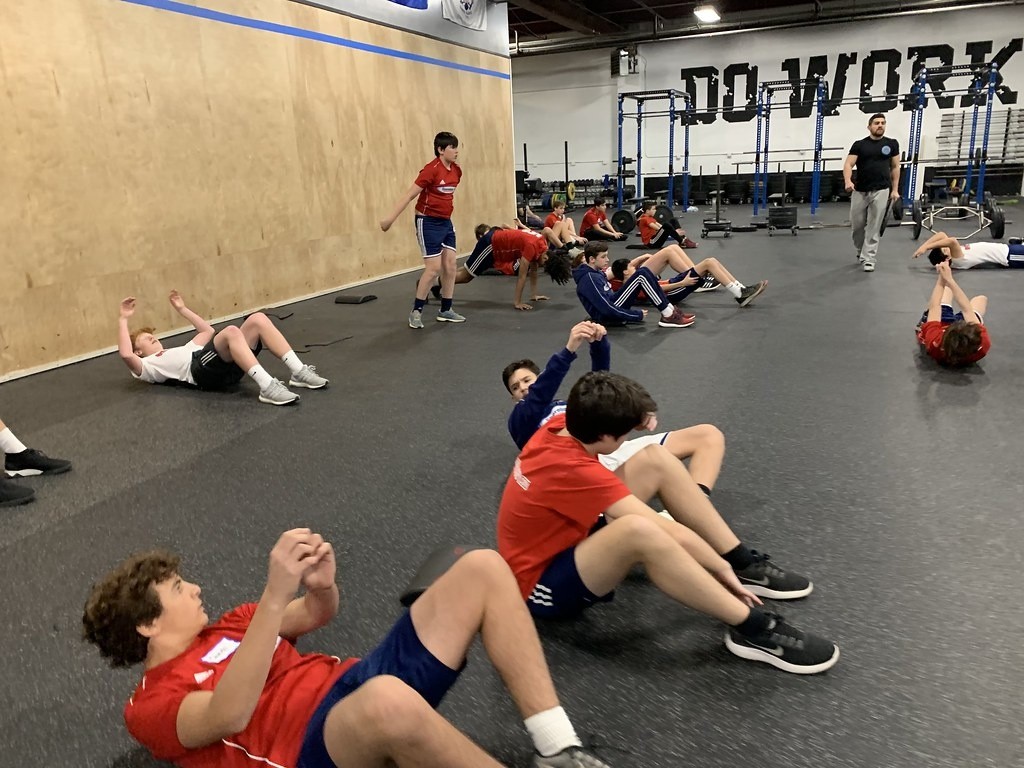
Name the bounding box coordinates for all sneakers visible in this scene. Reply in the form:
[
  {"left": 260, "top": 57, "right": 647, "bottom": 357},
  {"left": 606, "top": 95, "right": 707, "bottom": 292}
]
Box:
[
  {"left": 694, "top": 280, "right": 721, "bottom": 292},
  {"left": 0, "top": 478, "right": 35, "bottom": 505},
  {"left": 4, "top": 448, "right": 71, "bottom": 476},
  {"left": 258, "top": 377, "right": 300, "bottom": 405},
  {"left": 658, "top": 310, "right": 694, "bottom": 327},
  {"left": 702, "top": 276, "right": 716, "bottom": 281},
  {"left": 679, "top": 236, "right": 699, "bottom": 248},
  {"left": 715, "top": 549, "right": 814, "bottom": 599},
  {"left": 735, "top": 281, "right": 763, "bottom": 307},
  {"left": 408, "top": 309, "right": 424, "bottom": 329},
  {"left": 725, "top": 610, "right": 840, "bottom": 674},
  {"left": 436, "top": 307, "right": 466, "bottom": 322},
  {"left": 746, "top": 280, "right": 768, "bottom": 294},
  {"left": 532, "top": 737, "right": 616, "bottom": 768},
  {"left": 674, "top": 305, "right": 695, "bottom": 319},
  {"left": 288, "top": 364, "right": 328, "bottom": 388}
]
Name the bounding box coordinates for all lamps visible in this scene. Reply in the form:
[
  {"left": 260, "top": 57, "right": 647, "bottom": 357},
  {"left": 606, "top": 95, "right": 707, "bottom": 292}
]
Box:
[{"left": 693, "top": 0, "right": 722, "bottom": 23}]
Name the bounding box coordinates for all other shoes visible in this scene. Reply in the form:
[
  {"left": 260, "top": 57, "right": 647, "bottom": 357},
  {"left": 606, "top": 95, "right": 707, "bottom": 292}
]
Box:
[
  {"left": 430, "top": 276, "right": 442, "bottom": 299},
  {"left": 863, "top": 260, "right": 874, "bottom": 271}
]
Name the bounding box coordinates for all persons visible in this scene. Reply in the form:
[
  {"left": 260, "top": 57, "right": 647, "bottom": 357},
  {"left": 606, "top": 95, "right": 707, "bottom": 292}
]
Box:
[
  {"left": 545, "top": 201, "right": 588, "bottom": 246},
  {"left": 913, "top": 231, "right": 1024, "bottom": 269},
  {"left": 416, "top": 228, "right": 570, "bottom": 310},
  {"left": 573, "top": 241, "right": 768, "bottom": 327},
  {"left": 475, "top": 206, "right": 584, "bottom": 275},
  {"left": 640, "top": 202, "right": 697, "bottom": 248},
  {"left": 916, "top": 262, "right": 990, "bottom": 363},
  {"left": 85, "top": 527, "right": 608, "bottom": 768},
  {"left": 379, "top": 132, "right": 465, "bottom": 328},
  {"left": 503, "top": 320, "right": 725, "bottom": 516},
  {"left": 499, "top": 370, "right": 840, "bottom": 672},
  {"left": 844, "top": 114, "right": 901, "bottom": 271},
  {"left": 118, "top": 290, "right": 329, "bottom": 405},
  {"left": 0, "top": 420, "right": 73, "bottom": 506},
  {"left": 580, "top": 198, "right": 628, "bottom": 240}
]
